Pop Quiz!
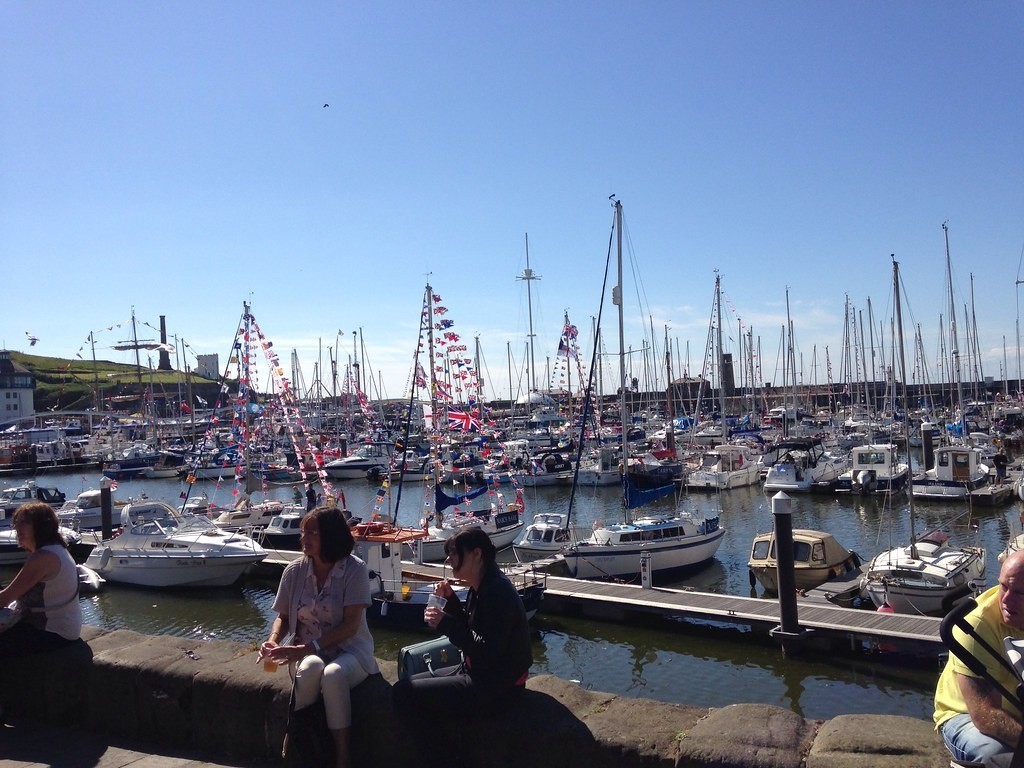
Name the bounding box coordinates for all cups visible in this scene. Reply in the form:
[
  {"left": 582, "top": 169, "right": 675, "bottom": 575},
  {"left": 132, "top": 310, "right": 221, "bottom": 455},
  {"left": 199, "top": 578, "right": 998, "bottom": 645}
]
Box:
[
  {"left": 264, "top": 648, "right": 279, "bottom": 673},
  {"left": 425, "top": 594, "right": 447, "bottom": 622}
]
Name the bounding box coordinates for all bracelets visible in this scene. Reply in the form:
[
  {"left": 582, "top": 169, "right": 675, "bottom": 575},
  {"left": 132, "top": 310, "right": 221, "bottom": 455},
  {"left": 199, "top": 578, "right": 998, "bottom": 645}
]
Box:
[{"left": 312, "top": 640, "right": 321, "bottom": 656}]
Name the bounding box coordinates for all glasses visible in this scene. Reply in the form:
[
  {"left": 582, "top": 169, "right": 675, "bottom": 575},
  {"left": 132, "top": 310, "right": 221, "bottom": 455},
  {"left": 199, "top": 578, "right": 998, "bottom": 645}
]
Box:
[{"left": 300, "top": 530, "right": 320, "bottom": 535}]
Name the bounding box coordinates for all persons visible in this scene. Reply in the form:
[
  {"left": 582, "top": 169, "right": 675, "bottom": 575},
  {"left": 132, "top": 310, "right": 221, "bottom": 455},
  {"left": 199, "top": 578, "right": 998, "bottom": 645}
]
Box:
[
  {"left": 993, "top": 449, "right": 1008, "bottom": 488},
  {"left": 932, "top": 548, "right": 1024, "bottom": 768},
  {"left": 256, "top": 507, "right": 378, "bottom": 768},
  {"left": 394, "top": 527, "right": 532, "bottom": 768},
  {"left": 647, "top": 439, "right": 653, "bottom": 450},
  {"left": 292, "top": 482, "right": 322, "bottom": 512},
  {"left": 77, "top": 448, "right": 82, "bottom": 463},
  {"left": 729, "top": 429, "right": 733, "bottom": 438},
  {"left": 103, "top": 461, "right": 121, "bottom": 469},
  {"left": 8, "top": 444, "right": 14, "bottom": 461},
  {"left": 114, "top": 526, "right": 124, "bottom": 538},
  {"left": 0, "top": 502, "right": 82, "bottom": 729}
]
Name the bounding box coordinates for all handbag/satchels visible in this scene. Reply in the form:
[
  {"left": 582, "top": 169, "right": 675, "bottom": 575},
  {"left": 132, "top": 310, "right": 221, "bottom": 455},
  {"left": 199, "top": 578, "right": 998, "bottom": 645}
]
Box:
[
  {"left": 282, "top": 727, "right": 314, "bottom": 760},
  {"left": 0, "top": 600, "right": 29, "bottom": 633},
  {"left": 398, "top": 636, "right": 464, "bottom": 680}
]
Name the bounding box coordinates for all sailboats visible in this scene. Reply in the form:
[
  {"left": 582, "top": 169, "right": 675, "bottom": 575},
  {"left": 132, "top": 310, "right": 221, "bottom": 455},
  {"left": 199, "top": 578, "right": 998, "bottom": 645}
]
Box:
[
  {"left": 562, "top": 194, "right": 726, "bottom": 585},
  {"left": 101, "top": 220, "right": 1024, "bottom": 508},
  {"left": 859, "top": 260, "right": 987, "bottom": 614},
  {"left": 385, "top": 272, "right": 523, "bottom": 564}
]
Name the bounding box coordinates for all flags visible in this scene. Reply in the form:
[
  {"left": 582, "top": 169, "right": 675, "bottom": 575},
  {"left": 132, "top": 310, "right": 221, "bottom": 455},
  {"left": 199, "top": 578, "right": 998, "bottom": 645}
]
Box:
[
  {"left": 444, "top": 403, "right": 481, "bottom": 431},
  {"left": 561, "top": 325, "right": 578, "bottom": 338},
  {"left": 557, "top": 340, "right": 577, "bottom": 358}
]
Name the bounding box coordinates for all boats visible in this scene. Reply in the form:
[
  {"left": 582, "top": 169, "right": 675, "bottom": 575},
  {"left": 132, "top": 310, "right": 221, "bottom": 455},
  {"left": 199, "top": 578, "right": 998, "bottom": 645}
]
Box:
[
  {"left": 0, "top": 476, "right": 170, "bottom": 531},
  {"left": 272, "top": 461, "right": 550, "bottom": 630},
  {"left": 747, "top": 529, "right": 861, "bottom": 593},
  {"left": 512, "top": 512, "right": 575, "bottom": 563},
  {"left": 176, "top": 292, "right": 351, "bottom": 551},
  {"left": 0, "top": 518, "right": 98, "bottom": 566},
  {"left": 83, "top": 497, "right": 269, "bottom": 590}
]
